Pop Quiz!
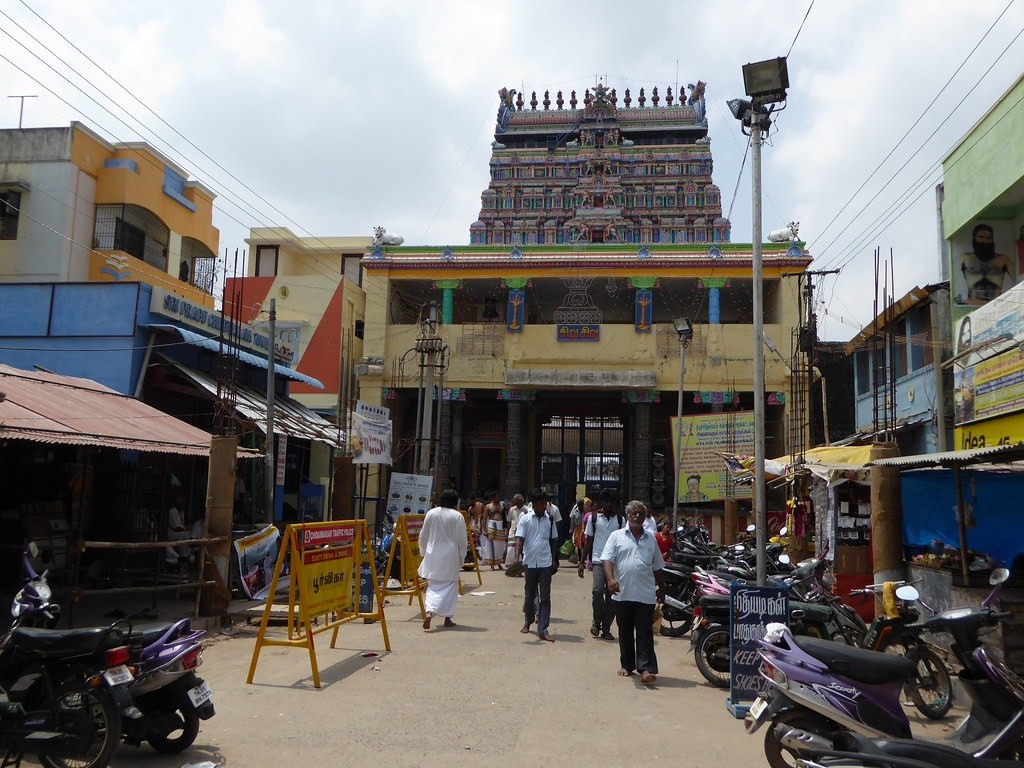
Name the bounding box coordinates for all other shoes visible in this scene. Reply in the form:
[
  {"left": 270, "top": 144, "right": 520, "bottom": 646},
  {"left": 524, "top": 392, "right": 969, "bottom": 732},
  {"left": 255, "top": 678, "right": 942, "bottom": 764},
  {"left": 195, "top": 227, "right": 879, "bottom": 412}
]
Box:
[{"left": 506, "top": 561, "right": 528, "bottom": 577}]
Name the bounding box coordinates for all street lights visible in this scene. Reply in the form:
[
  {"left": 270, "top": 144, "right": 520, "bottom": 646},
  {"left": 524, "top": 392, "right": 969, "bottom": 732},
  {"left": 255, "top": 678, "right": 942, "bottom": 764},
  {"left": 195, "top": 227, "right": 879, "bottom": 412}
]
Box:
[
  {"left": 673, "top": 317, "right": 695, "bottom": 533},
  {"left": 761, "top": 329, "right": 799, "bottom": 452},
  {"left": 724, "top": 57, "right": 790, "bottom": 593}
]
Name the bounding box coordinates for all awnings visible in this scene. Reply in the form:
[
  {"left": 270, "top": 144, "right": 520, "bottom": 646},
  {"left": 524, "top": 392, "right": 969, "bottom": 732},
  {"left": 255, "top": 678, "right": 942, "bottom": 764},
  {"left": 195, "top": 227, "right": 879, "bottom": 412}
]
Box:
[
  {"left": 139, "top": 321, "right": 327, "bottom": 390},
  {"left": 862, "top": 443, "right": 1015, "bottom": 469},
  {"left": 159, "top": 351, "right": 349, "bottom": 442},
  {"left": 1, "top": 364, "right": 266, "bottom": 459}
]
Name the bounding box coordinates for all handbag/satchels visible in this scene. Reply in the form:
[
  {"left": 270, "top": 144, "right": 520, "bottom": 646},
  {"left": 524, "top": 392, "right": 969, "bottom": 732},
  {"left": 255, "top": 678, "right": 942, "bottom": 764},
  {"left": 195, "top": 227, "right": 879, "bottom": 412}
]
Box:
[
  {"left": 652, "top": 605, "right": 664, "bottom": 635},
  {"left": 560, "top": 539, "right": 575, "bottom": 557}
]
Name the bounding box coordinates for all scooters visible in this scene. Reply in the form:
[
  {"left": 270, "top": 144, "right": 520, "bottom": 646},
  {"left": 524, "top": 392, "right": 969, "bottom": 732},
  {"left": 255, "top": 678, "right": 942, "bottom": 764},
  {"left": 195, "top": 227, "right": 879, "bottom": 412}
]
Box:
[{"left": 741, "top": 569, "right": 1024, "bottom": 768}]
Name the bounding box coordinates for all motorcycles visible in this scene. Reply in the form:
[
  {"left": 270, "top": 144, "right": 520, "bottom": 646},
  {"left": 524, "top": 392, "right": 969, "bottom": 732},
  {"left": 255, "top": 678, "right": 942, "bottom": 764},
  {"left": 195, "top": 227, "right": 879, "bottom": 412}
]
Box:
[
  {"left": 373, "top": 510, "right": 402, "bottom": 582},
  {"left": 463, "top": 531, "right": 482, "bottom": 571},
  {"left": 655, "top": 515, "right": 953, "bottom": 722},
  {"left": 10, "top": 542, "right": 216, "bottom": 754},
  {"left": 0, "top": 618, "right": 145, "bottom": 768}
]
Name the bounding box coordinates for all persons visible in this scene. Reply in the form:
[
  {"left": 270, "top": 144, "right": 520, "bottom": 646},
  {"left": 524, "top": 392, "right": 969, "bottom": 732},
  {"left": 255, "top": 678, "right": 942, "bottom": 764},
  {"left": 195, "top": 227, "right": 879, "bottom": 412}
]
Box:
[
  {"left": 189, "top": 507, "right": 205, "bottom": 575},
  {"left": 678, "top": 475, "right": 711, "bottom": 503},
  {"left": 576, "top": 488, "right": 627, "bottom": 640},
  {"left": 417, "top": 489, "right": 467, "bottom": 629},
  {"left": 600, "top": 500, "right": 667, "bottom": 683},
  {"left": 568, "top": 496, "right": 603, "bottom": 572},
  {"left": 465, "top": 492, "right": 563, "bottom": 572},
  {"left": 643, "top": 504, "right": 676, "bottom": 602},
  {"left": 953, "top": 224, "right": 1016, "bottom": 301},
  {"left": 167, "top": 497, "right": 188, "bottom": 532},
  {"left": 512, "top": 492, "right": 559, "bottom": 642}
]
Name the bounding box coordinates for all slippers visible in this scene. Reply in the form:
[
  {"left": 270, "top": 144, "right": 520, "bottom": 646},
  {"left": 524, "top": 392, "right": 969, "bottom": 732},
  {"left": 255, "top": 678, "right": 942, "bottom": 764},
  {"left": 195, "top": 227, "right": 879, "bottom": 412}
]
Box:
[
  {"left": 590, "top": 620, "right": 601, "bottom": 637},
  {"left": 600, "top": 632, "right": 615, "bottom": 640}
]
